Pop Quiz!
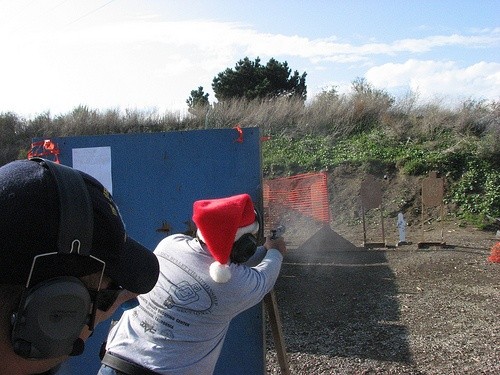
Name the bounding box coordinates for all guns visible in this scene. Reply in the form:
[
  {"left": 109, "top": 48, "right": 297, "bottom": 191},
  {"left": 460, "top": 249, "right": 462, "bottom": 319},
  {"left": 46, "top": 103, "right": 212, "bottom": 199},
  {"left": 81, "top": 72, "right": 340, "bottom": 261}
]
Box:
[{"left": 270, "top": 225, "right": 285, "bottom": 240}]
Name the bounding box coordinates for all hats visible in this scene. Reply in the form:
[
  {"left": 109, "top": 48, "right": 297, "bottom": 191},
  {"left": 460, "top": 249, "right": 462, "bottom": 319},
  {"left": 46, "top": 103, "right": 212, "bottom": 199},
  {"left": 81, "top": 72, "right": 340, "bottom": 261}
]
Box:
[
  {"left": 0, "top": 159, "right": 160, "bottom": 295},
  {"left": 192, "top": 194, "right": 260, "bottom": 284}
]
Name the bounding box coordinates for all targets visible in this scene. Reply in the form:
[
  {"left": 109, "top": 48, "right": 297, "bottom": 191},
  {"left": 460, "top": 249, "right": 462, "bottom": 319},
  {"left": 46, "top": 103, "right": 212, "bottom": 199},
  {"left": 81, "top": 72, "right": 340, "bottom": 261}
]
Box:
[
  {"left": 360, "top": 175, "right": 386, "bottom": 248},
  {"left": 396, "top": 213, "right": 412, "bottom": 246},
  {"left": 417, "top": 172, "right": 447, "bottom": 250}
]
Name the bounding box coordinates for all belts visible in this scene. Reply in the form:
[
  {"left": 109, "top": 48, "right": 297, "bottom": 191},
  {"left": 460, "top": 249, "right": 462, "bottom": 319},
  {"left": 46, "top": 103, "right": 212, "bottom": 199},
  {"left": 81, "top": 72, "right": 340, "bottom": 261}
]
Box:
[{"left": 101, "top": 353, "right": 156, "bottom": 375}]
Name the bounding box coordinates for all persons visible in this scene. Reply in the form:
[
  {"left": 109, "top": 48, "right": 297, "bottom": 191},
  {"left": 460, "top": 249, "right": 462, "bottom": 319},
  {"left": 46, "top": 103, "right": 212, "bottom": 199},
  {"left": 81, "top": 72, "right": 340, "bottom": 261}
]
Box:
[
  {"left": 0, "top": 157, "right": 161, "bottom": 375},
  {"left": 98, "top": 193, "right": 286, "bottom": 375}
]
